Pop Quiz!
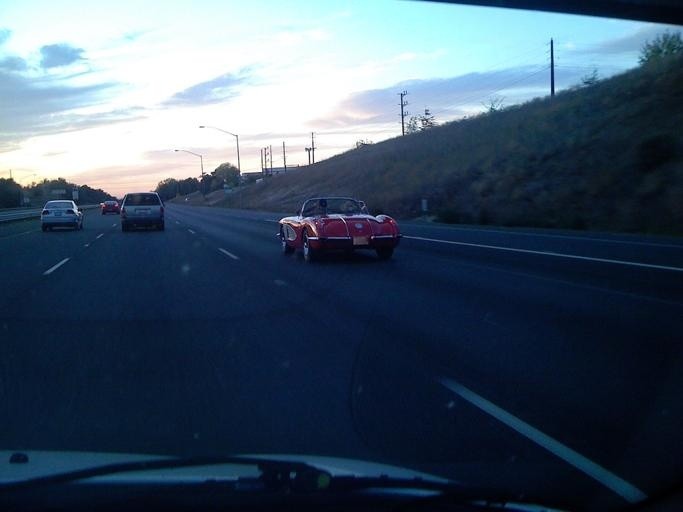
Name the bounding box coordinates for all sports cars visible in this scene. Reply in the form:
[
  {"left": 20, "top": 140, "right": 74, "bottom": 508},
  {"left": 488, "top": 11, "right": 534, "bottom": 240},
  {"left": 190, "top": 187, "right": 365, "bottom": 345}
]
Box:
[{"left": 277, "top": 197, "right": 402, "bottom": 260}]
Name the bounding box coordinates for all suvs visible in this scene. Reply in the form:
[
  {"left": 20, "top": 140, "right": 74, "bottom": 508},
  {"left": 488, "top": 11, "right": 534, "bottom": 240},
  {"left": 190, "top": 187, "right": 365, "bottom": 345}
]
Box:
[{"left": 121, "top": 192, "right": 165, "bottom": 231}]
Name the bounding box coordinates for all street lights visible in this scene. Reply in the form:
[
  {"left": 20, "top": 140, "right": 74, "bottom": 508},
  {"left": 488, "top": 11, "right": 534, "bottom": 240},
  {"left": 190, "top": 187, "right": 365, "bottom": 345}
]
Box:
[
  {"left": 200, "top": 126, "right": 240, "bottom": 177},
  {"left": 175, "top": 150, "right": 203, "bottom": 178}
]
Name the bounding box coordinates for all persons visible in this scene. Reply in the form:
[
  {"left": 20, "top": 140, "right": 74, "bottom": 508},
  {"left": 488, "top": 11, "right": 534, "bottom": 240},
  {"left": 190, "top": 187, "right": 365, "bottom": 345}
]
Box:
[
  {"left": 341, "top": 201, "right": 353, "bottom": 211},
  {"left": 317, "top": 200, "right": 328, "bottom": 210}
]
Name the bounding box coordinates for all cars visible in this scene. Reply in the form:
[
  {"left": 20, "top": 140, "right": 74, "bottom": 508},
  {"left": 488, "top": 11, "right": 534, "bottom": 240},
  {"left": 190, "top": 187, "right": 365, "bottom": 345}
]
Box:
[
  {"left": 101, "top": 201, "right": 120, "bottom": 215},
  {"left": 41, "top": 200, "right": 84, "bottom": 229}
]
